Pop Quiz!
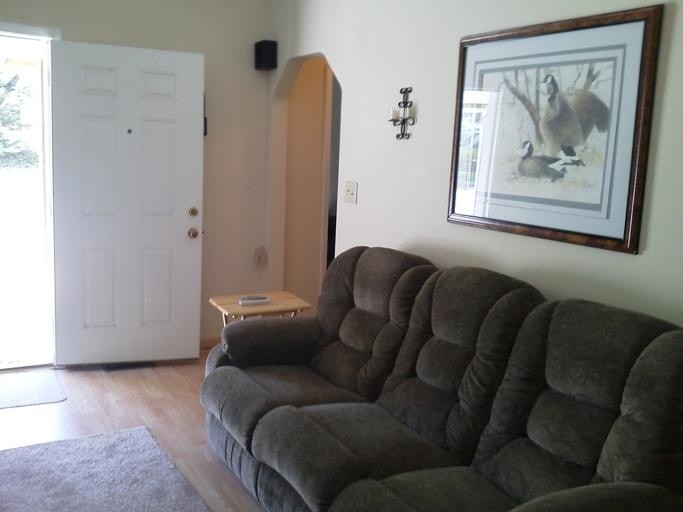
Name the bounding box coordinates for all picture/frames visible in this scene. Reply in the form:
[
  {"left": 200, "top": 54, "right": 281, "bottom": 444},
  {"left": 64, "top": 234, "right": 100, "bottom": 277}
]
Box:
[{"left": 447, "top": 4, "right": 664, "bottom": 254}]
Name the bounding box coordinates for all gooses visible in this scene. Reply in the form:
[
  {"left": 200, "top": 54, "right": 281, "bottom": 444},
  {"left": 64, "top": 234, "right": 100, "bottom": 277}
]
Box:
[
  {"left": 517, "top": 140, "right": 564, "bottom": 179},
  {"left": 537, "top": 74, "right": 610, "bottom": 167}
]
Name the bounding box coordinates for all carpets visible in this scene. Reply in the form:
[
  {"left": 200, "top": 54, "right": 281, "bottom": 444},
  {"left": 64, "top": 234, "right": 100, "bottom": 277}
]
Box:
[
  {"left": 0, "top": 426, "right": 212, "bottom": 512},
  {"left": 0, "top": 369, "right": 68, "bottom": 409}
]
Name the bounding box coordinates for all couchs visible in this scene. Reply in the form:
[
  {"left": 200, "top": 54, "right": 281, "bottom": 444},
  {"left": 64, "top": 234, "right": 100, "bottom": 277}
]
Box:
[{"left": 199, "top": 246, "right": 683, "bottom": 511}]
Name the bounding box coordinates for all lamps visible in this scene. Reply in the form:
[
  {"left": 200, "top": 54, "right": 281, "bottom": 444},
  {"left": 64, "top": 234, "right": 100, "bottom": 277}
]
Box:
[{"left": 390, "top": 87, "right": 418, "bottom": 140}]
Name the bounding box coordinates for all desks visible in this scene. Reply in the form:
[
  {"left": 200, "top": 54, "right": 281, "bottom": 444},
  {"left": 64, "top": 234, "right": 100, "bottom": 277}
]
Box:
[{"left": 208, "top": 291, "right": 312, "bottom": 328}]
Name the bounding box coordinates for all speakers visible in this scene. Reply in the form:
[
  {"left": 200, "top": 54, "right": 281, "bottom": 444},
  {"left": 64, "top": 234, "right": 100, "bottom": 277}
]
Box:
[{"left": 255, "top": 39, "right": 277, "bottom": 70}]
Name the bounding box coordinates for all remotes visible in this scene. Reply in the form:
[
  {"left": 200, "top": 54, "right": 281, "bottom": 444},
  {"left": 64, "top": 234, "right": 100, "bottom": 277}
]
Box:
[{"left": 238, "top": 295, "right": 271, "bottom": 304}]
